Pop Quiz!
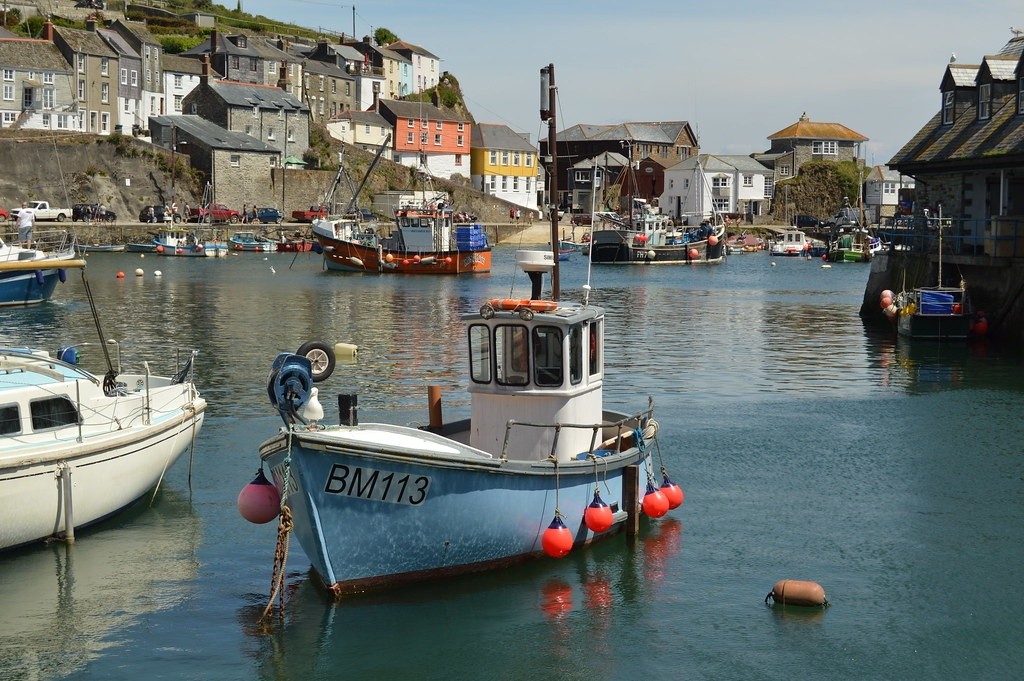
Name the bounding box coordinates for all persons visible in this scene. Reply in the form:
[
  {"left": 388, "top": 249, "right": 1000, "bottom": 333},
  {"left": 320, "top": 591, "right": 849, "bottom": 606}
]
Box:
[
  {"left": 163, "top": 203, "right": 205, "bottom": 224},
  {"left": 510, "top": 207, "right": 534, "bottom": 224},
  {"left": 700, "top": 213, "right": 741, "bottom": 244},
  {"left": 16, "top": 202, "right": 35, "bottom": 249},
  {"left": 241, "top": 204, "right": 249, "bottom": 224},
  {"left": 86, "top": 203, "right": 106, "bottom": 223},
  {"left": 252, "top": 205, "right": 260, "bottom": 224},
  {"left": 147, "top": 205, "right": 155, "bottom": 223}
]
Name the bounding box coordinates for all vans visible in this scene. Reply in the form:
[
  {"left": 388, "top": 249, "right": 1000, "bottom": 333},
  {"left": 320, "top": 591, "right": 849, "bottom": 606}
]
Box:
[{"left": 571, "top": 212, "right": 621, "bottom": 226}]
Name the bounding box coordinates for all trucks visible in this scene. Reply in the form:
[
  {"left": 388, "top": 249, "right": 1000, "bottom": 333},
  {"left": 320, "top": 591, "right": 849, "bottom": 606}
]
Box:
[
  {"left": 827, "top": 210, "right": 871, "bottom": 229},
  {"left": 292, "top": 205, "right": 330, "bottom": 223},
  {"left": 10, "top": 200, "right": 73, "bottom": 222}
]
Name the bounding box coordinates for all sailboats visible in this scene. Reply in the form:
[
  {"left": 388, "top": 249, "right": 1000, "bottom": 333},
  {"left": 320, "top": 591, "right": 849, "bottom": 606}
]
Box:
[
  {"left": 899, "top": 203, "right": 979, "bottom": 340},
  {"left": 0, "top": 0, "right": 208, "bottom": 553},
  {"left": 851, "top": 172, "right": 882, "bottom": 256},
  {"left": 590, "top": 123, "right": 728, "bottom": 265}
]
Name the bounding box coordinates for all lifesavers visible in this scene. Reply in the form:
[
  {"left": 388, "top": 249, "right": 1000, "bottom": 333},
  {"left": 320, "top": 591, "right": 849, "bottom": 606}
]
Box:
[
  {"left": 489, "top": 298, "right": 559, "bottom": 311},
  {"left": 927, "top": 208, "right": 938, "bottom": 231}
]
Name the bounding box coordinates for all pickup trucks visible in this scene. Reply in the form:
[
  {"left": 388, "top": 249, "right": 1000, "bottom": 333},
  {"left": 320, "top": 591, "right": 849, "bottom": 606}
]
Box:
[{"left": 189, "top": 203, "right": 240, "bottom": 224}]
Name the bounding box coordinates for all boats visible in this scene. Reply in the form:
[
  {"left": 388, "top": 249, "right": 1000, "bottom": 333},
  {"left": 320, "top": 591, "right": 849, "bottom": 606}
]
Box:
[
  {"left": 259, "top": 62, "right": 660, "bottom": 597},
  {"left": 72, "top": 214, "right": 313, "bottom": 258},
  {"left": 724, "top": 184, "right": 865, "bottom": 264},
  {"left": 0, "top": 230, "right": 77, "bottom": 306},
  {"left": 309, "top": 131, "right": 491, "bottom": 273}
]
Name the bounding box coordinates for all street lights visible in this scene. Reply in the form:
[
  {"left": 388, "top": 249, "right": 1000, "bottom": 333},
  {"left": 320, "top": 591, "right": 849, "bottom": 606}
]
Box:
[{"left": 172, "top": 141, "right": 186, "bottom": 204}]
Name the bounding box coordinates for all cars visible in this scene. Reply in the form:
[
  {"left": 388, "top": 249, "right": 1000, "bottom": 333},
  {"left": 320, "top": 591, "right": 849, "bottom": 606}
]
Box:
[
  {"left": 790, "top": 214, "right": 826, "bottom": 228},
  {"left": 239, "top": 208, "right": 285, "bottom": 224},
  {"left": 0, "top": 209, "right": 9, "bottom": 223},
  {"left": 139, "top": 204, "right": 181, "bottom": 223},
  {"left": 72, "top": 203, "right": 118, "bottom": 223}
]
